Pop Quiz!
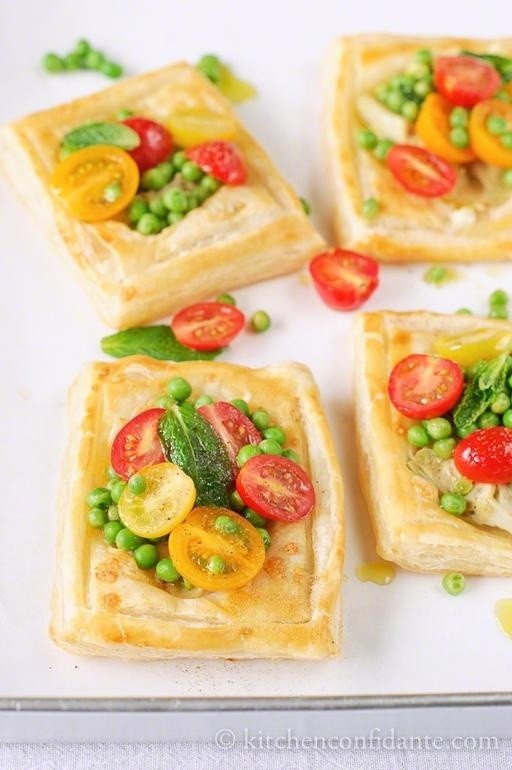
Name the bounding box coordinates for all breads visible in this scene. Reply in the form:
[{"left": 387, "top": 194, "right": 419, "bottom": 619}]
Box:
[{"left": 3, "top": 31, "right": 512, "bottom": 663}]
